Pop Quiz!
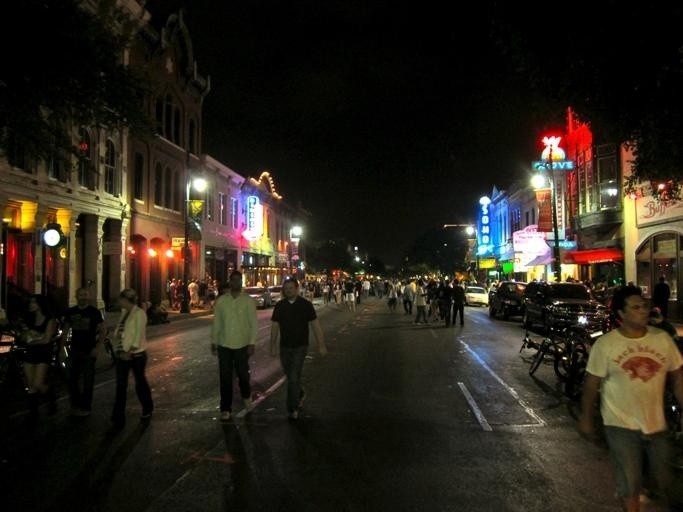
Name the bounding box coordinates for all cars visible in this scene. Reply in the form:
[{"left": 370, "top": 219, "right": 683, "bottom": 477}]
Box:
[
  {"left": 490, "top": 279, "right": 527, "bottom": 320},
  {"left": 264, "top": 284, "right": 282, "bottom": 308},
  {"left": 0, "top": 308, "right": 115, "bottom": 394},
  {"left": 242, "top": 285, "right": 273, "bottom": 310},
  {"left": 461, "top": 284, "right": 490, "bottom": 307}
]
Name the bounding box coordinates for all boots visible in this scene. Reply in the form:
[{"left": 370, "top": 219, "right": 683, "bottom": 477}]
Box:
[
  {"left": 45, "top": 384, "right": 57, "bottom": 416},
  {"left": 21, "top": 391, "right": 40, "bottom": 426}
]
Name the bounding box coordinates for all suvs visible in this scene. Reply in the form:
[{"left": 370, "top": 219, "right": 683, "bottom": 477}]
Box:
[{"left": 517, "top": 279, "right": 610, "bottom": 338}]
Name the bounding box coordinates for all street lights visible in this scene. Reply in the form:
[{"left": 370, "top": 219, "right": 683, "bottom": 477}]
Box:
[
  {"left": 527, "top": 166, "right": 563, "bottom": 283},
  {"left": 175, "top": 168, "right": 210, "bottom": 315}
]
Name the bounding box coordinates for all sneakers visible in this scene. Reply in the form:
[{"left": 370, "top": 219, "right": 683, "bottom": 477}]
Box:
[
  {"left": 62, "top": 408, "right": 90, "bottom": 417},
  {"left": 288, "top": 408, "right": 298, "bottom": 426},
  {"left": 139, "top": 408, "right": 154, "bottom": 418},
  {"left": 296, "top": 391, "right": 307, "bottom": 409},
  {"left": 221, "top": 411, "right": 232, "bottom": 421},
  {"left": 110, "top": 417, "right": 126, "bottom": 432},
  {"left": 242, "top": 399, "right": 251, "bottom": 408}
]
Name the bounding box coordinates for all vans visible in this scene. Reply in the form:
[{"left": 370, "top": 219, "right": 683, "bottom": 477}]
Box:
[{"left": 301, "top": 279, "right": 320, "bottom": 297}]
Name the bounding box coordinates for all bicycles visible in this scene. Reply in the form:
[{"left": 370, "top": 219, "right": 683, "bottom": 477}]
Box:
[{"left": 517, "top": 305, "right": 682, "bottom": 474}]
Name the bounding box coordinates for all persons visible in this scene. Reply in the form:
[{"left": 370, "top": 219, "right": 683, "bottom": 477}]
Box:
[
  {"left": 653, "top": 276, "right": 670, "bottom": 311},
  {"left": 20, "top": 294, "right": 59, "bottom": 424},
  {"left": 582, "top": 286, "right": 683, "bottom": 511},
  {"left": 299, "top": 268, "right": 477, "bottom": 326},
  {"left": 210, "top": 272, "right": 259, "bottom": 423},
  {"left": 169, "top": 276, "right": 220, "bottom": 314},
  {"left": 108, "top": 290, "right": 154, "bottom": 425},
  {"left": 58, "top": 286, "right": 106, "bottom": 413},
  {"left": 649, "top": 307, "right": 663, "bottom": 325},
  {"left": 268, "top": 280, "right": 327, "bottom": 419}
]
